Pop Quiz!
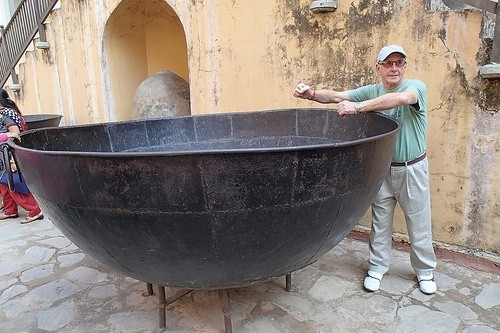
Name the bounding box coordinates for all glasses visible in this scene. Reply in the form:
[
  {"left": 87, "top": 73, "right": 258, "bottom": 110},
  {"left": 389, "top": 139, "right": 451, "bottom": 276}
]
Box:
[{"left": 378, "top": 59, "right": 406, "bottom": 67}]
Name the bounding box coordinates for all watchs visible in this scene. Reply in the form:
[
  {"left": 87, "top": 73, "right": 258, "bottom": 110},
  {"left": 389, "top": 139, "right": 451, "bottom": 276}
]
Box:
[{"left": 354, "top": 101, "right": 362, "bottom": 114}]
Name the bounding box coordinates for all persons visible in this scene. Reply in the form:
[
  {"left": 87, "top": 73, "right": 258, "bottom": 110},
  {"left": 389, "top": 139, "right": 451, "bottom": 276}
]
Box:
[
  {"left": 293, "top": 44, "right": 437, "bottom": 293},
  {"left": 0, "top": 88, "right": 44, "bottom": 223}
]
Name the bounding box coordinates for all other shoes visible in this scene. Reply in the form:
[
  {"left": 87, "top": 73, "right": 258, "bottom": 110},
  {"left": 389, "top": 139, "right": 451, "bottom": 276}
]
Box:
[
  {"left": 0, "top": 214, "right": 18, "bottom": 220},
  {"left": 20, "top": 211, "right": 44, "bottom": 224}
]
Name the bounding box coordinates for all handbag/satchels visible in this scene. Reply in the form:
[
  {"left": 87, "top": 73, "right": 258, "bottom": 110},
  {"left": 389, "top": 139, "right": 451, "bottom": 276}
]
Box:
[{"left": 0, "top": 170, "right": 30, "bottom": 195}]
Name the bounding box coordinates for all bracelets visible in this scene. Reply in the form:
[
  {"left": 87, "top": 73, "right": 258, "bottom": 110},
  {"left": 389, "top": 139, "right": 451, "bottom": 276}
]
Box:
[{"left": 308, "top": 89, "right": 315, "bottom": 99}]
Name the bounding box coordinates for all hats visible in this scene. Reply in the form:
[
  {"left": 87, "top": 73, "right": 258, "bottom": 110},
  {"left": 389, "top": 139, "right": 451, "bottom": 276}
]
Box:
[{"left": 377, "top": 45, "right": 406, "bottom": 64}]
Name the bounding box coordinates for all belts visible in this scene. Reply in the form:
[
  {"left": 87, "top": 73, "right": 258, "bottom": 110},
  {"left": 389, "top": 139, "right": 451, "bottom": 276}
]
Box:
[{"left": 391, "top": 152, "right": 427, "bottom": 166}]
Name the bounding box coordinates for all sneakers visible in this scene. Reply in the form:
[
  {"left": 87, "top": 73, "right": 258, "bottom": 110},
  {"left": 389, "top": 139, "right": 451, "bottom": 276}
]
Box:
[
  {"left": 363, "top": 269, "right": 380, "bottom": 291},
  {"left": 416, "top": 274, "right": 437, "bottom": 294}
]
